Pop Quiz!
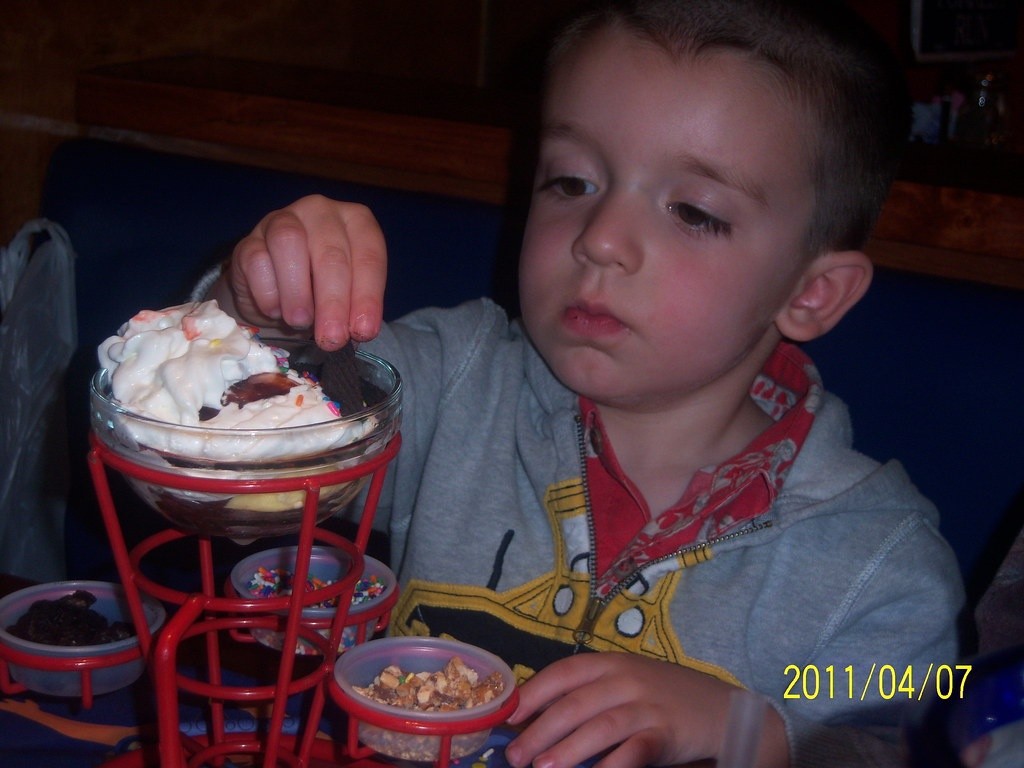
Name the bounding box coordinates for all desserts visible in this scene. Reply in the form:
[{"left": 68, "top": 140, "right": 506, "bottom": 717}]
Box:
[{"left": 93, "top": 299, "right": 387, "bottom": 535}]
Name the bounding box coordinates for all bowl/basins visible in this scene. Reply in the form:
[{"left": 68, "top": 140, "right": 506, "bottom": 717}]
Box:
[
  {"left": 333, "top": 635, "right": 519, "bottom": 758},
  {"left": 91, "top": 347, "right": 403, "bottom": 537},
  {"left": 0, "top": 580, "right": 166, "bottom": 697},
  {"left": 230, "top": 545, "right": 397, "bottom": 656}
]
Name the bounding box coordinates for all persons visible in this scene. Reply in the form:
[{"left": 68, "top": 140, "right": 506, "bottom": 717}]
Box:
[{"left": 184, "top": 0, "right": 965, "bottom": 767}]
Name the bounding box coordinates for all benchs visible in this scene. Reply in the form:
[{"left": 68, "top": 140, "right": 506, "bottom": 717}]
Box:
[{"left": 36, "top": 133, "right": 1024, "bottom": 657}]
